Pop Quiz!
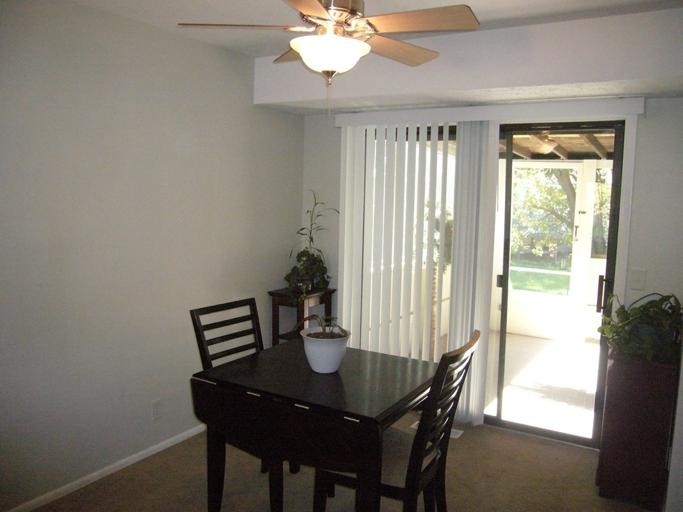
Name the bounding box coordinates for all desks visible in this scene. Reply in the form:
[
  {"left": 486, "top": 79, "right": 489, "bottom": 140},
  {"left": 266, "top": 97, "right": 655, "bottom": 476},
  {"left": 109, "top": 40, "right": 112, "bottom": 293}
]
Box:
[
  {"left": 189, "top": 340, "right": 439, "bottom": 512},
  {"left": 266, "top": 287, "right": 337, "bottom": 350}
]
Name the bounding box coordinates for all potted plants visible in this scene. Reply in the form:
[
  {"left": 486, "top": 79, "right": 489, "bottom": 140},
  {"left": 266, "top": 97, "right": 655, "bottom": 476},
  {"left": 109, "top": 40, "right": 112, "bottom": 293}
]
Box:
[
  {"left": 284, "top": 189, "right": 341, "bottom": 295},
  {"left": 291, "top": 313, "right": 351, "bottom": 374}
]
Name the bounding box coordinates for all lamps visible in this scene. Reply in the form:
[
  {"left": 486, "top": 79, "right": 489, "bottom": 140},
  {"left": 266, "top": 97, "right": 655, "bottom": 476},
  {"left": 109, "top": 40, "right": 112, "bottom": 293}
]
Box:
[{"left": 289, "top": 1, "right": 374, "bottom": 88}]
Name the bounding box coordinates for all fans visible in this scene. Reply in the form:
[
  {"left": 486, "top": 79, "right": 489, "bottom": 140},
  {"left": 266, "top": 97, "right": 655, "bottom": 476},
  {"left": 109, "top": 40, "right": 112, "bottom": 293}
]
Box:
[{"left": 175, "top": 1, "right": 481, "bottom": 69}]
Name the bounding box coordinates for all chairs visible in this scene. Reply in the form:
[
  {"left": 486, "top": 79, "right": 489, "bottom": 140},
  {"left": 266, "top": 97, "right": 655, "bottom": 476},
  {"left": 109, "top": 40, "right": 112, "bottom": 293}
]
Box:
[
  {"left": 188, "top": 298, "right": 274, "bottom": 475},
  {"left": 327, "top": 327, "right": 480, "bottom": 512}
]
